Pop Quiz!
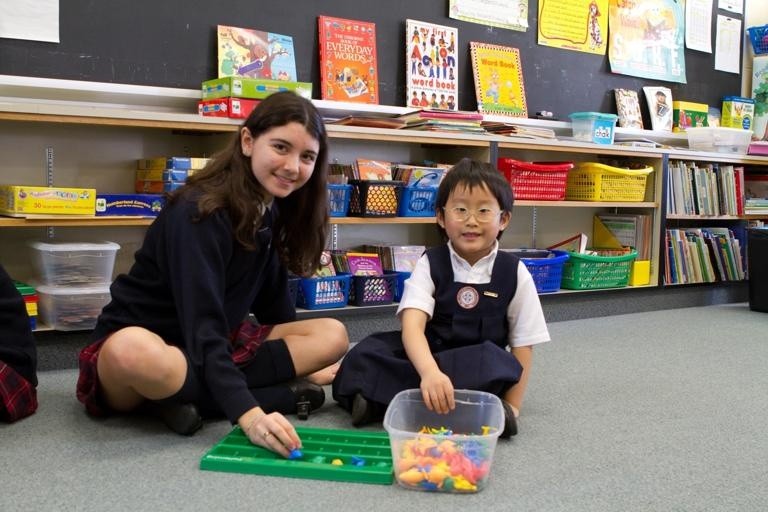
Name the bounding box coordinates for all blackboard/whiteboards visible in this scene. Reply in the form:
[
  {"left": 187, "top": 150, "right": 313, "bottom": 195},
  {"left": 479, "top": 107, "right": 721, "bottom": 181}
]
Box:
[{"left": 0, "top": 1, "right": 746, "bottom": 127}]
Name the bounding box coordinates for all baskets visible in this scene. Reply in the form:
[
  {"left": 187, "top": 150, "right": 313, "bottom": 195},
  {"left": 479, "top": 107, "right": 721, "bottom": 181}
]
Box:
[
  {"left": 347, "top": 178, "right": 405, "bottom": 217},
  {"left": 520, "top": 249, "right": 570, "bottom": 294},
  {"left": 324, "top": 182, "right": 354, "bottom": 217},
  {"left": 298, "top": 272, "right": 351, "bottom": 310},
  {"left": 561, "top": 246, "right": 638, "bottom": 290},
  {"left": 497, "top": 156, "right": 576, "bottom": 201},
  {"left": 287, "top": 273, "right": 300, "bottom": 310},
  {"left": 347, "top": 270, "right": 400, "bottom": 306},
  {"left": 393, "top": 272, "right": 411, "bottom": 303},
  {"left": 401, "top": 186, "right": 440, "bottom": 217},
  {"left": 566, "top": 158, "right": 655, "bottom": 202}
]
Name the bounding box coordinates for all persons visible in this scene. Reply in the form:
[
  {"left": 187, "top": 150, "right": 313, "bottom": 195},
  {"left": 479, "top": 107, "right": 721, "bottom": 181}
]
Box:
[
  {"left": 333, "top": 156, "right": 552, "bottom": 440},
  {"left": 0, "top": 264, "right": 39, "bottom": 424},
  {"left": 74, "top": 86, "right": 354, "bottom": 460}
]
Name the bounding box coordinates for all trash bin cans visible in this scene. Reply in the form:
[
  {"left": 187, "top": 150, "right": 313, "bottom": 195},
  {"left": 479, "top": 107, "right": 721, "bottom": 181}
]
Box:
[{"left": 746, "top": 228, "right": 768, "bottom": 312}]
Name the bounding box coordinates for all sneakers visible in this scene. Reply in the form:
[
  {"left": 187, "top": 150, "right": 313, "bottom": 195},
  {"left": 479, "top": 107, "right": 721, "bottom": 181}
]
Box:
[
  {"left": 350, "top": 395, "right": 385, "bottom": 427},
  {"left": 498, "top": 401, "right": 519, "bottom": 438}
]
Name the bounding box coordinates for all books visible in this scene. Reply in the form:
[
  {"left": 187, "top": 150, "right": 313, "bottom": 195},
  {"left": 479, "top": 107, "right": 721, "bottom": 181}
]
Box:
[
  {"left": 663, "top": 159, "right": 768, "bottom": 288},
  {"left": 318, "top": 13, "right": 376, "bottom": 105},
  {"left": 619, "top": 136, "right": 688, "bottom": 151},
  {"left": 642, "top": 85, "right": 675, "bottom": 133},
  {"left": 497, "top": 214, "right": 652, "bottom": 290},
  {"left": 319, "top": 108, "right": 560, "bottom": 142},
  {"left": 301, "top": 159, "right": 460, "bottom": 303},
  {"left": 405, "top": 17, "right": 460, "bottom": 111}
]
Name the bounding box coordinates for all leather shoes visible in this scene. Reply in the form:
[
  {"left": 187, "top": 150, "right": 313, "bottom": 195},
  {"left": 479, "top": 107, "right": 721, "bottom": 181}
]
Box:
[
  {"left": 272, "top": 381, "right": 325, "bottom": 419},
  {"left": 160, "top": 401, "right": 204, "bottom": 436}
]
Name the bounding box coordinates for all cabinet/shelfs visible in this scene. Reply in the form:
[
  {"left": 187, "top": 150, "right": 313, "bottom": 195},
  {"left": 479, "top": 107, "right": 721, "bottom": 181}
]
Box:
[{"left": 0, "top": 72, "right": 768, "bottom": 345}]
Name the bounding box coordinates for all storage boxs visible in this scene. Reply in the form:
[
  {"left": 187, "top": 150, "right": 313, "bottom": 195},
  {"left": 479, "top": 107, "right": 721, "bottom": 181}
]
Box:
[
  {"left": 25, "top": 238, "right": 121, "bottom": 288},
  {"left": 568, "top": 112, "right": 618, "bottom": 144},
  {"left": 26, "top": 280, "right": 112, "bottom": 330},
  {"left": 672, "top": 96, "right": 756, "bottom": 155},
  {"left": 382, "top": 389, "right": 506, "bottom": 494}
]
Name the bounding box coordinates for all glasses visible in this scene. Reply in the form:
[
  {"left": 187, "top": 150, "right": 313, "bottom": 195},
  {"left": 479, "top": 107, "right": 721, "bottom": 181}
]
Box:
[{"left": 442, "top": 205, "right": 505, "bottom": 223}]
